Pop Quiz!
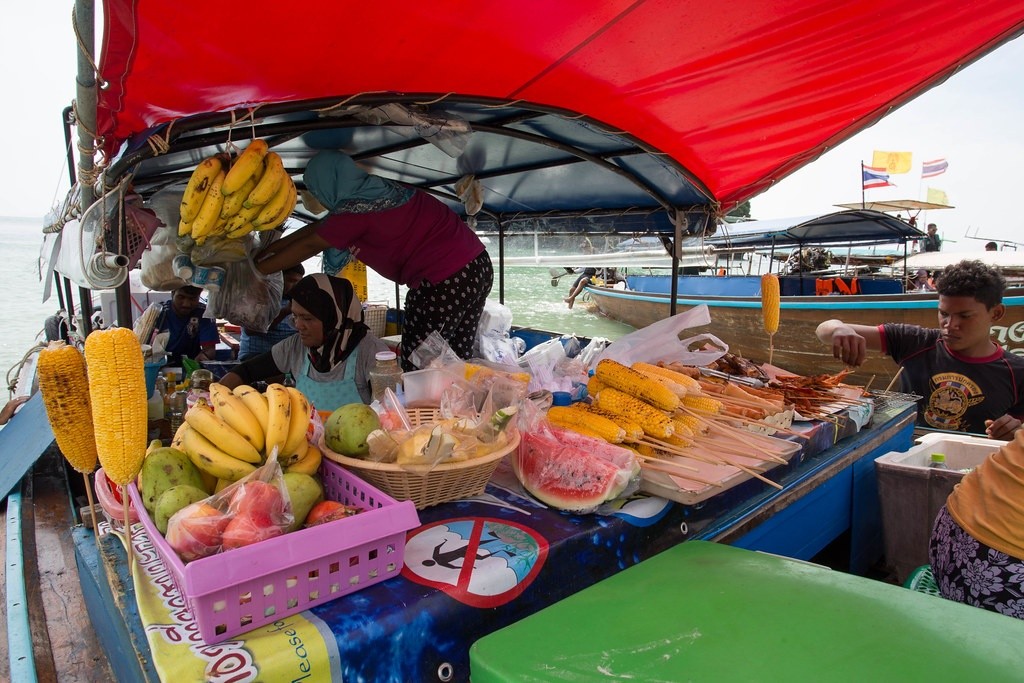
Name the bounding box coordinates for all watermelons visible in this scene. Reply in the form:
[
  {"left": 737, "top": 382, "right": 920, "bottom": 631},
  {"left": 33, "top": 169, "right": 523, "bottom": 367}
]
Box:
[{"left": 511, "top": 430, "right": 639, "bottom": 510}]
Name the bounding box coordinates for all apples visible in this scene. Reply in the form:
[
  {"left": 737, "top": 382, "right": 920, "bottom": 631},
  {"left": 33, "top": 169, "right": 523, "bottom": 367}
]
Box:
[
  {"left": 308, "top": 500, "right": 349, "bottom": 525},
  {"left": 166, "top": 481, "right": 282, "bottom": 562}
]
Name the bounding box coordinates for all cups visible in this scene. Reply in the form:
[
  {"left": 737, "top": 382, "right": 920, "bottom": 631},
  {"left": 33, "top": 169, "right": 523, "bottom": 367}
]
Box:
[
  {"left": 206, "top": 266, "right": 226, "bottom": 293},
  {"left": 191, "top": 264, "right": 211, "bottom": 288},
  {"left": 172, "top": 254, "right": 193, "bottom": 280}
]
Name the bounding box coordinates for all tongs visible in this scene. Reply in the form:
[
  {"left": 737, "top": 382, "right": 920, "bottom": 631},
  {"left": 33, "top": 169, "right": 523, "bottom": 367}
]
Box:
[{"left": 698, "top": 366, "right": 764, "bottom": 387}]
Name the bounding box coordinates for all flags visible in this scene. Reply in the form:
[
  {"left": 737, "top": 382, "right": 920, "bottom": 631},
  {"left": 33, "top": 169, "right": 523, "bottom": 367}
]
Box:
[
  {"left": 873, "top": 151, "right": 912, "bottom": 175},
  {"left": 921, "top": 159, "right": 948, "bottom": 179},
  {"left": 862, "top": 165, "right": 892, "bottom": 189},
  {"left": 927, "top": 188, "right": 949, "bottom": 205}
]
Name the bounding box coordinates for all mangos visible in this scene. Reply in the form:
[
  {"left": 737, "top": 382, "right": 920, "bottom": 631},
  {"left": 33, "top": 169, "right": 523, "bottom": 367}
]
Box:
[
  {"left": 155, "top": 485, "right": 209, "bottom": 535},
  {"left": 324, "top": 403, "right": 380, "bottom": 457},
  {"left": 141, "top": 448, "right": 204, "bottom": 509},
  {"left": 269, "top": 473, "right": 321, "bottom": 533}
]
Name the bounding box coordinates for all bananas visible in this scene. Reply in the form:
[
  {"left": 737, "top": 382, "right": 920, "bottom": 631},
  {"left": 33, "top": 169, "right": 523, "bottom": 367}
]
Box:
[
  {"left": 170, "top": 383, "right": 322, "bottom": 480},
  {"left": 178, "top": 140, "right": 296, "bottom": 262}
]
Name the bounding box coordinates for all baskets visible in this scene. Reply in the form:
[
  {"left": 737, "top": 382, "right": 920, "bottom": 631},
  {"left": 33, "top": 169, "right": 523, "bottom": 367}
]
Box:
[
  {"left": 318, "top": 408, "right": 521, "bottom": 510},
  {"left": 97, "top": 455, "right": 421, "bottom": 645}
]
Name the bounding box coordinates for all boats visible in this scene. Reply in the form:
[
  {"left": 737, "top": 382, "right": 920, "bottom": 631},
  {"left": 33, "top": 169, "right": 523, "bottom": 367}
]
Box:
[
  {"left": 0, "top": 0, "right": 1024, "bottom": 681},
  {"left": 582, "top": 208, "right": 1023, "bottom": 388}
]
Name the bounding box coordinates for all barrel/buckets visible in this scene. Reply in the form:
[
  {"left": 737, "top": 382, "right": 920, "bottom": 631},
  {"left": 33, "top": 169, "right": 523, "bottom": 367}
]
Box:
[{"left": 144, "top": 362, "right": 161, "bottom": 399}]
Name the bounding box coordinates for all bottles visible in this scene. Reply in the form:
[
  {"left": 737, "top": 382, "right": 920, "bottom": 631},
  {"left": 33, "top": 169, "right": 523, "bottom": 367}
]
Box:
[
  {"left": 163, "top": 372, "right": 176, "bottom": 421},
  {"left": 369, "top": 351, "right": 404, "bottom": 403},
  {"left": 920, "top": 284, "right": 927, "bottom": 293},
  {"left": 927, "top": 453, "right": 950, "bottom": 469},
  {"left": 170, "top": 385, "right": 188, "bottom": 440},
  {"left": 157, "top": 371, "right": 164, "bottom": 397},
  {"left": 148, "top": 387, "right": 165, "bottom": 421}
]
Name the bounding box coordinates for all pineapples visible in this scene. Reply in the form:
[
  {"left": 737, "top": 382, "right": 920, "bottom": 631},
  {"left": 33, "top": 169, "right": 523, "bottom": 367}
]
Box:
[{"left": 397, "top": 426, "right": 510, "bottom": 464}]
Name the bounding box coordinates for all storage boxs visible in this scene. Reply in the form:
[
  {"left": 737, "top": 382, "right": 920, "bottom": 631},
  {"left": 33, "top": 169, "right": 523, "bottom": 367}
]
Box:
[{"left": 95, "top": 201, "right": 161, "bottom": 271}]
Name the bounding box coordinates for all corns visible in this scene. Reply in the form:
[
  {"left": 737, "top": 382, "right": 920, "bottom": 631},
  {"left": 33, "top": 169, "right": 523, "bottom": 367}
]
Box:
[
  {"left": 761, "top": 274, "right": 780, "bottom": 335},
  {"left": 37, "top": 339, "right": 96, "bottom": 472},
  {"left": 547, "top": 359, "right": 724, "bottom": 458},
  {"left": 84, "top": 327, "right": 148, "bottom": 487}
]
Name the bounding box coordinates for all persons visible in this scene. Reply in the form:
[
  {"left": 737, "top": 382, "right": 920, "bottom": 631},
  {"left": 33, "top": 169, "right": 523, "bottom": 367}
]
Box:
[
  {"left": 985, "top": 242, "right": 998, "bottom": 251},
  {"left": 905, "top": 269, "right": 938, "bottom": 291},
  {"left": 215, "top": 273, "right": 404, "bottom": 412},
  {"left": 239, "top": 264, "right": 305, "bottom": 361},
  {"left": 153, "top": 283, "right": 220, "bottom": 365},
  {"left": 255, "top": 149, "right": 494, "bottom": 373},
  {"left": 601, "top": 267, "right": 630, "bottom": 291},
  {"left": 814, "top": 258, "right": 1024, "bottom": 441},
  {"left": 928, "top": 425, "right": 1024, "bottom": 621},
  {"left": 920, "top": 223, "right": 941, "bottom": 252},
  {"left": 563, "top": 265, "right": 608, "bottom": 308}
]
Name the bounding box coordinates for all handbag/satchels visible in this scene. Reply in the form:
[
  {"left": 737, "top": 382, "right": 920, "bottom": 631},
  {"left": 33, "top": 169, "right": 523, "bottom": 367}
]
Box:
[
  {"left": 202, "top": 236, "right": 283, "bottom": 333},
  {"left": 594, "top": 303, "right": 729, "bottom": 373}
]
